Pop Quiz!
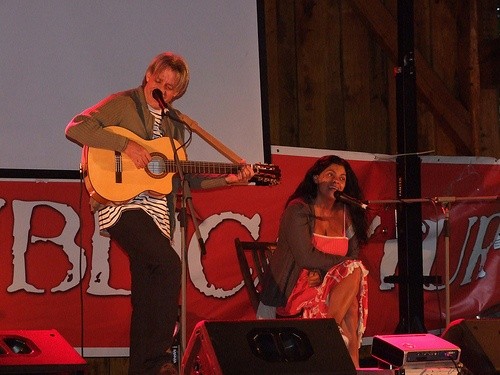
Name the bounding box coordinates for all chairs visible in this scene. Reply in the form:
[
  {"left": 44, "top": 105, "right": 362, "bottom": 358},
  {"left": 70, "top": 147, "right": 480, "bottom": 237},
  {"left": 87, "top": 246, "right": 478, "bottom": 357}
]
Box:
[{"left": 235, "top": 236, "right": 279, "bottom": 315}]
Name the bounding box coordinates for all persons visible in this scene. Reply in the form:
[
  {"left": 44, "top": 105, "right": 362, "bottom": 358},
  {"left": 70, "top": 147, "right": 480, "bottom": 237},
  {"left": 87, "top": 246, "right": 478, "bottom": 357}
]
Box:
[
  {"left": 256, "top": 155, "right": 369, "bottom": 368},
  {"left": 66, "top": 52, "right": 254, "bottom": 375}
]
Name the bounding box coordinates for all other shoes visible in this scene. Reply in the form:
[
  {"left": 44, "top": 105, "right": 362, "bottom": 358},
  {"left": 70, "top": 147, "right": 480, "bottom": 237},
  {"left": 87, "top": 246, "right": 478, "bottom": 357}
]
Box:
[{"left": 159, "top": 363, "right": 178, "bottom": 375}]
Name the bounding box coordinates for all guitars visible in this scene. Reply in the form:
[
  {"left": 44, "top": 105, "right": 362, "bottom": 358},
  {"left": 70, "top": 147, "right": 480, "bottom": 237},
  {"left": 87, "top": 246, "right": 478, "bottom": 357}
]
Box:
[{"left": 81, "top": 125, "right": 283, "bottom": 207}]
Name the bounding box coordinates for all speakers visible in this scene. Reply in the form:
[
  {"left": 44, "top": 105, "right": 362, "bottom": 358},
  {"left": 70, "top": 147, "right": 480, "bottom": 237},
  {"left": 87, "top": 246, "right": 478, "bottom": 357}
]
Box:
[
  {"left": 447, "top": 318, "right": 500, "bottom": 374},
  {"left": 0, "top": 329, "right": 90, "bottom": 375},
  {"left": 179, "top": 317, "right": 358, "bottom": 375}
]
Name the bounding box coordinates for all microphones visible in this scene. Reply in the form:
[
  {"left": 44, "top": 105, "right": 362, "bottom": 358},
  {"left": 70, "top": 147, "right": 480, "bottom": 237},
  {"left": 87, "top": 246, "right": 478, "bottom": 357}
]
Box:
[
  {"left": 334, "top": 190, "right": 368, "bottom": 210},
  {"left": 152, "top": 89, "right": 169, "bottom": 114}
]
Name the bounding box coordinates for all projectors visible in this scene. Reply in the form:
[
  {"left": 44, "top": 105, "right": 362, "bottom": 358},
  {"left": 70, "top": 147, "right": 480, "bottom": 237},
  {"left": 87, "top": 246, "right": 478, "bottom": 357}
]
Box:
[{"left": 371, "top": 334, "right": 461, "bottom": 370}]
